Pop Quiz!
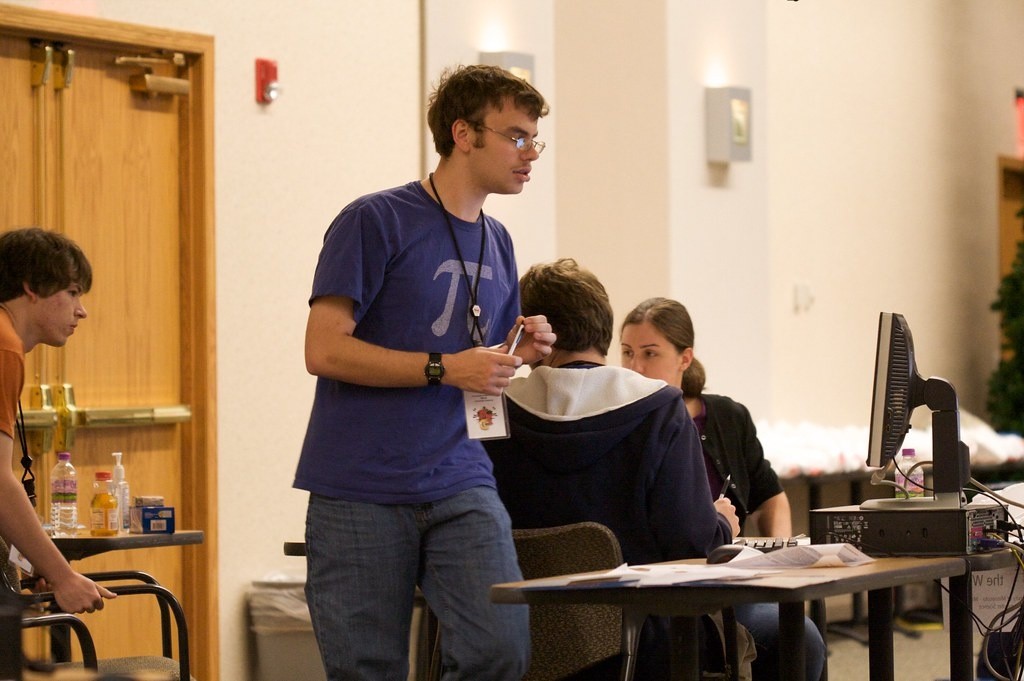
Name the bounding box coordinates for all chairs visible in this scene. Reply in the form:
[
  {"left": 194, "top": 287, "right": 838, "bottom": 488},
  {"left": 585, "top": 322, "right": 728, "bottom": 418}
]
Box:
[
  {"left": 1, "top": 569, "right": 195, "bottom": 680},
  {"left": 430, "top": 525, "right": 646, "bottom": 680}
]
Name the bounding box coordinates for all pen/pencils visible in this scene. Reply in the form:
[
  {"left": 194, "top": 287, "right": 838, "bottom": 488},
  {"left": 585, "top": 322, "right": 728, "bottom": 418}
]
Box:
[
  {"left": 507, "top": 320, "right": 525, "bottom": 355},
  {"left": 719, "top": 474, "right": 730, "bottom": 499}
]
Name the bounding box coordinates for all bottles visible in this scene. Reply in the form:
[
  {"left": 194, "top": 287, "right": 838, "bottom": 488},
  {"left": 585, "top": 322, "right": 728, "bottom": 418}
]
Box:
[
  {"left": 90, "top": 472, "right": 120, "bottom": 535},
  {"left": 51, "top": 453, "right": 78, "bottom": 537},
  {"left": 895, "top": 448, "right": 924, "bottom": 498}
]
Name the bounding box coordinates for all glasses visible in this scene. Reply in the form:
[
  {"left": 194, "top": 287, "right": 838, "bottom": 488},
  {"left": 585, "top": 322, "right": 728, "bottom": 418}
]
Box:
[{"left": 471, "top": 123, "right": 545, "bottom": 155}]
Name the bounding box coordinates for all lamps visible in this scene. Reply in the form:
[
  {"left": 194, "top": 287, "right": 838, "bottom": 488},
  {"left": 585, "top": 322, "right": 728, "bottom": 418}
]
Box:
[
  {"left": 703, "top": 85, "right": 756, "bottom": 168},
  {"left": 252, "top": 59, "right": 284, "bottom": 104},
  {"left": 476, "top": 51, "right": 533, "bottom": 86}
]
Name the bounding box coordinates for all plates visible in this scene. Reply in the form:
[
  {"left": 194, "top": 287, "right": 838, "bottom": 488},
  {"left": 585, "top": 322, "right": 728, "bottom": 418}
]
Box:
[{"left": 41, "top": 525, "right": 87, "bottom": 535}]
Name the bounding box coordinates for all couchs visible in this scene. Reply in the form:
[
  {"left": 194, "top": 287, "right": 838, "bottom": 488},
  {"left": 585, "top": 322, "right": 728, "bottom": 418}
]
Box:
[{"left": 248, "top": 585, "right": 421, "bottom": 681}]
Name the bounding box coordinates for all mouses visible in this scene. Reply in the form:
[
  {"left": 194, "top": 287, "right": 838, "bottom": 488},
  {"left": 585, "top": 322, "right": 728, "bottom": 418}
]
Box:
[{"left": 707, "top": 544, "right": 764, "bottom": 565}]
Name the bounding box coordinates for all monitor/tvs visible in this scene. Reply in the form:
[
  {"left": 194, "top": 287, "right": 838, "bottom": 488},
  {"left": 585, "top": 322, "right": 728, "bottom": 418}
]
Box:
[{"left": 858, "top": 312, "right": 970, "bottom": 511}]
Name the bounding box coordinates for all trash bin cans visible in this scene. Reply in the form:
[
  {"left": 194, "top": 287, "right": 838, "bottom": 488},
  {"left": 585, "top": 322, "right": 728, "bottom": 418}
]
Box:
[{"left": 248, "top": 577, "right": 328, "bottom": 681}]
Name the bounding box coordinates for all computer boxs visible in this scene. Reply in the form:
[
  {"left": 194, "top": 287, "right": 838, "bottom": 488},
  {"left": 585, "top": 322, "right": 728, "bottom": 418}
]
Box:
[{"left": 807, "top": 503, "right": 1009, "bottom": 555}]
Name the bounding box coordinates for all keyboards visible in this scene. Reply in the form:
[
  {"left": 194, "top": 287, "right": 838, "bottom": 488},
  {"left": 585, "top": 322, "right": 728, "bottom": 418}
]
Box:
[{"left": 733, "top": 538, "right": 799, "bottom": 555}]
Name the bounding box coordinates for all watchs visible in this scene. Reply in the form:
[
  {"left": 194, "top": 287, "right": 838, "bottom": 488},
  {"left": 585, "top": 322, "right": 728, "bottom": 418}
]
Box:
[{"left": 423, "top": 351, "right": 445, "bottom": 390}]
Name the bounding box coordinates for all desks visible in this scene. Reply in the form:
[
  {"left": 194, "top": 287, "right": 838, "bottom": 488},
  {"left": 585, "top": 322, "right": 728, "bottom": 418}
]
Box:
[
  {"left": 775, "top": 461, "right": 1024, "bottom": 650},
  {"left": 286, "top": 509, "right": 740, "bottom": 681},
  {"left": 489, "top": 557, "right": 966, "bottom": 681},
  {"left": 947, "top": 547, "right": 1023, "bottom": 681},
  {"left": 27, "top": 530, "right": 203, "bottom": 665}
]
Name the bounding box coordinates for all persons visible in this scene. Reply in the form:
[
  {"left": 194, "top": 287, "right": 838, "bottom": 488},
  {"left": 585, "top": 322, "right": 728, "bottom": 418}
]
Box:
[
  {"left": 304, "top": 64, "right": 558, "bottom": 681},
  {"left": 0, "top": 228, "right": 118, "bottom": 681},
  {"left": 479, "top": 257, "right": 826, "bottom": 681}
]
